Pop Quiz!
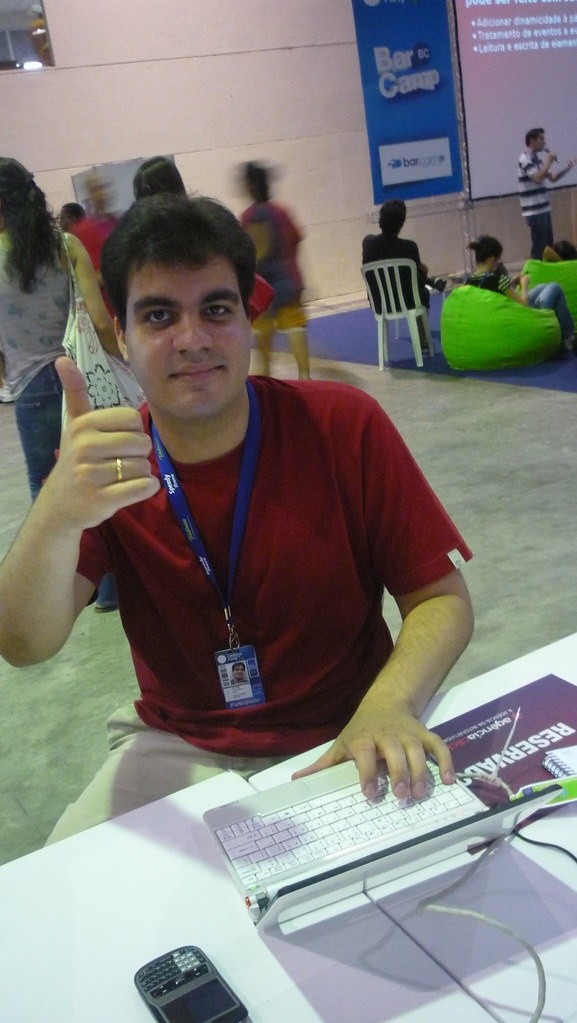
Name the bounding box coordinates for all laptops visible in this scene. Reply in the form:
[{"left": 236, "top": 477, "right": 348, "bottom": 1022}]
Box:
[{"left": 203, "top": 755, "right": 562, "bottom": 935}]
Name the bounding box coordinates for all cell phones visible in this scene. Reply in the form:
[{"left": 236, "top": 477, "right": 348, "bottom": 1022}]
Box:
[{"left": 134, "top": 945, "right": 249, "bottom": 1023}]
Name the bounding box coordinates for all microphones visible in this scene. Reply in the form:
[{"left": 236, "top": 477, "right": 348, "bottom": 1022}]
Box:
[{"left": 544, "top": 148, "right": 560, "bottom": 163}]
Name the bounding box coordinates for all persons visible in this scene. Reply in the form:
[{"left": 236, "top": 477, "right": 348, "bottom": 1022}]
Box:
[
  {"left": 1, "top": 157, "right": 126, "bottom": 506},
  {"left": 517, "top": 128, "right": 577, "bottom": 259},
  {"left": 230, "top": 662, "right": 248, "bottom": 684},
  {"left": 59, "top": 156, "right": 189, "bottom": 319},
  {"left": 466, "top": 234, "right": 575, "bottom": 351},
  {"left": 0, "top": 197, "right": 476, "bottom": 851},
  {"left": 553, "top": 240, "right": 577, "bottom": 261},
  {"left": 237, "top": 162, "right": 311, "bottom": 380},
  {"left": 360, "top": 200, "right": 447, "bottom": 356}
]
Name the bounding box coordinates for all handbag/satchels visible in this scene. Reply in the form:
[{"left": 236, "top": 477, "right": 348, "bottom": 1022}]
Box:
[{"left": 61, "top": 232, "right": 148, "bottom": 440}]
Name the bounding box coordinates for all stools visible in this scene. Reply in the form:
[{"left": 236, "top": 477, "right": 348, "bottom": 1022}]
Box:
[
  {"left": 440, "top": 284, "right": 561, "bottom": 370},
  {"left": 515, "top": 259, "right": 577, "bottom": 332}
]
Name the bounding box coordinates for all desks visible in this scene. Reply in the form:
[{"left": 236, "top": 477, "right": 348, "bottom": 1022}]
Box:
[
  {"left": 249, "top": 631, "right": 577, "bottom": 1022},
  {"left": 0, "top": 772, "right": 498, "bottom": 1023}
]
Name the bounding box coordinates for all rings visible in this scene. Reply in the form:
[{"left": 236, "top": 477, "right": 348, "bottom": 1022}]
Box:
[{"left": 115, "top": 458, "right": 122, "bottom": 481}]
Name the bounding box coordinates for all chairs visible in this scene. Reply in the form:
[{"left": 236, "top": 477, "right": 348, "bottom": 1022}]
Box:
[{"left": 361, "top": 258, "right": 435, "bottom": 371}]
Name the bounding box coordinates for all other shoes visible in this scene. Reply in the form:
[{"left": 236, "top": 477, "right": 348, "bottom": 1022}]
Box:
[
  {"left": 422, "top": 344, "right": 429, "bottom": 356},
  {"left": 435, "top": 279, "right": 447, "bottom": 293}
]
